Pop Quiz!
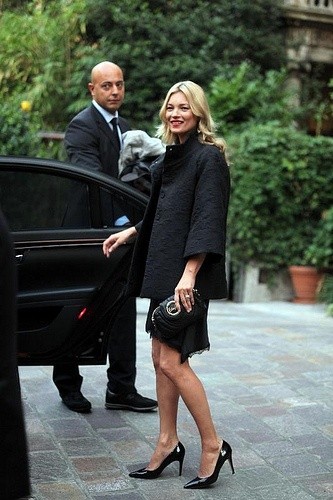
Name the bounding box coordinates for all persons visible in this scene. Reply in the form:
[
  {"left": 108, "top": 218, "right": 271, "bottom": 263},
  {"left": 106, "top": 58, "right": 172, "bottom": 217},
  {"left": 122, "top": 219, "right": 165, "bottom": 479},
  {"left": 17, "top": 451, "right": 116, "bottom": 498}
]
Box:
[
  {"left": 53, "top": 62, "right": 158, "bottom": 414},
  {"left": 103, "top": 80, "right": 236, "bottom": 489}
]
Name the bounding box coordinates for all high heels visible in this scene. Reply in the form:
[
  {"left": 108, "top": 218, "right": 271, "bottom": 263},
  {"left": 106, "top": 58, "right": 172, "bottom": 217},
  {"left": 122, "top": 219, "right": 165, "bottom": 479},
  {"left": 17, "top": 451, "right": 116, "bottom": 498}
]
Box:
[
  {"left": 128, "top": 441, "right": 186, "bottom": 480},
  {"left": 184, "top": 439, "right": 235, "bottom": 489}
]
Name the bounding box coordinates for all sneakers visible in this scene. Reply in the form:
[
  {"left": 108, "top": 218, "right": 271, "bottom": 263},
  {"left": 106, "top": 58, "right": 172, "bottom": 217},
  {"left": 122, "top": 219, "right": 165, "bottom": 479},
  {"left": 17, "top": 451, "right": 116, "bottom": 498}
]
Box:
[
  {"left": 60, "top": 390, "right": 92, "bottom": 412},
  {"left": 104, "top": 388, "right": 158, "bottom": 412}
]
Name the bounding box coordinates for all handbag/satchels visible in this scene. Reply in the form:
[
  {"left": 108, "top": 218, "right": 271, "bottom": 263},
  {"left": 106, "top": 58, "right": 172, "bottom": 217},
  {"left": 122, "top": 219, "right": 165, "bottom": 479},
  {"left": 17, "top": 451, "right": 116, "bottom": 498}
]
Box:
[{"left": 152, "top": 288, "right": 207, "bottom": 343}]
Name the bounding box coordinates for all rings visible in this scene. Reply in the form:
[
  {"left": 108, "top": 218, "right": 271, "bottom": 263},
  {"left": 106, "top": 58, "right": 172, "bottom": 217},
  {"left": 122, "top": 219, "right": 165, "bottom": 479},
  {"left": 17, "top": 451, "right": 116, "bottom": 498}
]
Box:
[{"left": 185, "top": 295, "right": 190, "bottom": 299}]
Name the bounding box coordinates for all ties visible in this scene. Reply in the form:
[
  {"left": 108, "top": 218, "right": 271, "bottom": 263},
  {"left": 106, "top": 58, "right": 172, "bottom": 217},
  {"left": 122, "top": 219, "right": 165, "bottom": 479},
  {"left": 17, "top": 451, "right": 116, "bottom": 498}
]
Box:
[{"left": 109, "top": 117, "right": 122, "bottom": 153}]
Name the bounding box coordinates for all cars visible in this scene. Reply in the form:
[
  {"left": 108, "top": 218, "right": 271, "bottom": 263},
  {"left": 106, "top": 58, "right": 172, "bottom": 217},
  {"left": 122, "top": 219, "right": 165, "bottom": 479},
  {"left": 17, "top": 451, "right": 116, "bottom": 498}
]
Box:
[{"left": 1, "top": 150, "right": 155, "bottom": 367}]
